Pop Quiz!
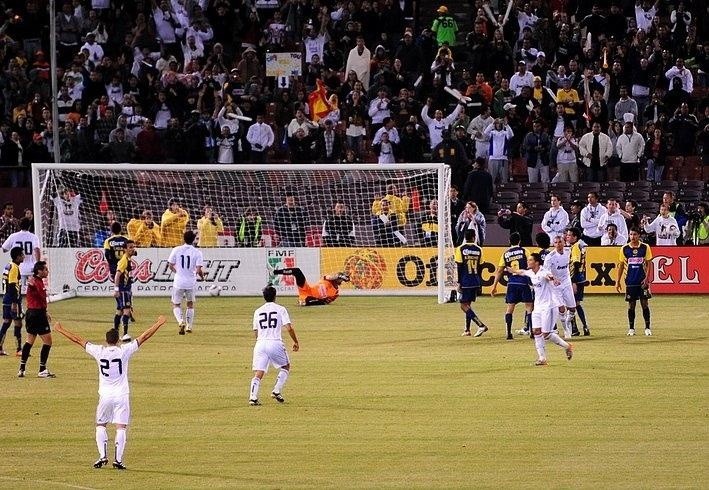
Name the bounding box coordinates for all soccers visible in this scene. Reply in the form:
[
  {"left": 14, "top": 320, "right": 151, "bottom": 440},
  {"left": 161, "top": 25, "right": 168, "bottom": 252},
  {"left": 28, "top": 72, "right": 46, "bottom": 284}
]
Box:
[{"left": 209, "top": 285, "right": 221, "bottom": 297}]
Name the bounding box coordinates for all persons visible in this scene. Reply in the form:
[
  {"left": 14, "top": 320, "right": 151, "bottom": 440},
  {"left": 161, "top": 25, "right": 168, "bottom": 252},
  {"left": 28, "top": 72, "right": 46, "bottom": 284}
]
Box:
[
  {"left": 542, "top": 235, "right": 578, "bottom": 338},
  {"left": 266, "top": 262, "right": 351, "bottom": 306},
  {"left": 103, "top": 221, "right": 135, "bottom": 322},
  {"left": 2, "top": 216, "right": 41, "bottom": 320},
  {"left": 507, "top": 253, "right": 573, "bottom": 365},
  {"left": 566, "top": 226, "right": 590, "bottom": 336},
  {"left": 113, "top": 240, "right": 135, "bottom": 342},
  {"left": 615, "top": 226, "right": 654, "bottom": 336},
  {"left": 491, "top": 231, "right": 535, "bottom": 339},
  {"left": 53, "top": 315, "right": 168, "bottom": 469},
  {"left": 167, "top": 230, "right": 205, "bottom": 335},
  {"left": 2, "top": 1, "right": 709, "bottom": 247},
  {"left": 535, "top": 233, "right": 551, "bottom": 265},
  {"left": 17, "top": 261, "right": 56, "bottom": 378},
  {"left": 248, "top": 286, "right": 299, "bottom": 406},
  {"left": 0, "top": 247, "right": 32, "bottom": 356},
  {"left": 456, "top": 229, "right": 488, "bottom": 338}
]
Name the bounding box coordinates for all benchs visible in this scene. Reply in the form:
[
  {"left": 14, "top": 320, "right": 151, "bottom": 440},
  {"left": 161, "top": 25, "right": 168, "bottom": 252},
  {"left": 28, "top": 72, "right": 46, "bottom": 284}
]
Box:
[{"left": 485, "top": 157, "right": 709, "bottom": 224}]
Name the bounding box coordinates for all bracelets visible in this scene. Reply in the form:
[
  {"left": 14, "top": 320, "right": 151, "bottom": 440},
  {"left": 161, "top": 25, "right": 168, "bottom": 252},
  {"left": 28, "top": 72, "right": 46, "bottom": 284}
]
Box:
[{"left": 114, "top": 286, "right": 120, "bottom": 293}]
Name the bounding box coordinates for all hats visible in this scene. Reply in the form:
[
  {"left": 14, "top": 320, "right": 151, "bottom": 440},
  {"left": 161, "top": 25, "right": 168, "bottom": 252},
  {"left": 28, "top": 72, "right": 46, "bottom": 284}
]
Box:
[
  {"left": 502, "top": 102, "right": 516, "bottom": 112},
  {"left": 536, "top": 51, "right": 546, "bottom": 58}
]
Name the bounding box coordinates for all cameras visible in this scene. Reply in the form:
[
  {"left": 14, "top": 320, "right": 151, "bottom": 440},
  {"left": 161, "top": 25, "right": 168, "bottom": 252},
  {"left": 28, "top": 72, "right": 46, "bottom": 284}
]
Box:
[{"left": 501, "top": 209, "right": 505, "bottom": 213}]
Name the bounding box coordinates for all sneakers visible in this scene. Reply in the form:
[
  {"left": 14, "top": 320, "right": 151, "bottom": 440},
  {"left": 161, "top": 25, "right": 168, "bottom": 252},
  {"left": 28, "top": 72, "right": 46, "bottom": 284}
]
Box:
[
  {"left": 248, "top": 398, "right": 260, "bottom": 406},
  {"left": 120, "top": 334, "right": 131, "bottom": 341},
  {"left": 460, "top": 325, "right": 590, "bottom": 366},
  {"left": 644, "top": 328, "right": 652, "bottom": 335},
  {"left": 264, "top": 262, "right": 276, "bottom": 278},
  {"left": 626, "top": 329, "right": 636, "bottom": 336},
  {"left": 271, "top": 391, "right": 285, "bottom": 403},
  {"left": 178, "top": 322, "right": 186, "bottom": 335},
  {"left": 92, "top": 457, "right": 109, "bottom": 469},
  {"left": 113, "top": 460, "right": 126, "bottom": 469},
  {"left": 0, "top": 350, "right": 56, "bottom": 378}
]
